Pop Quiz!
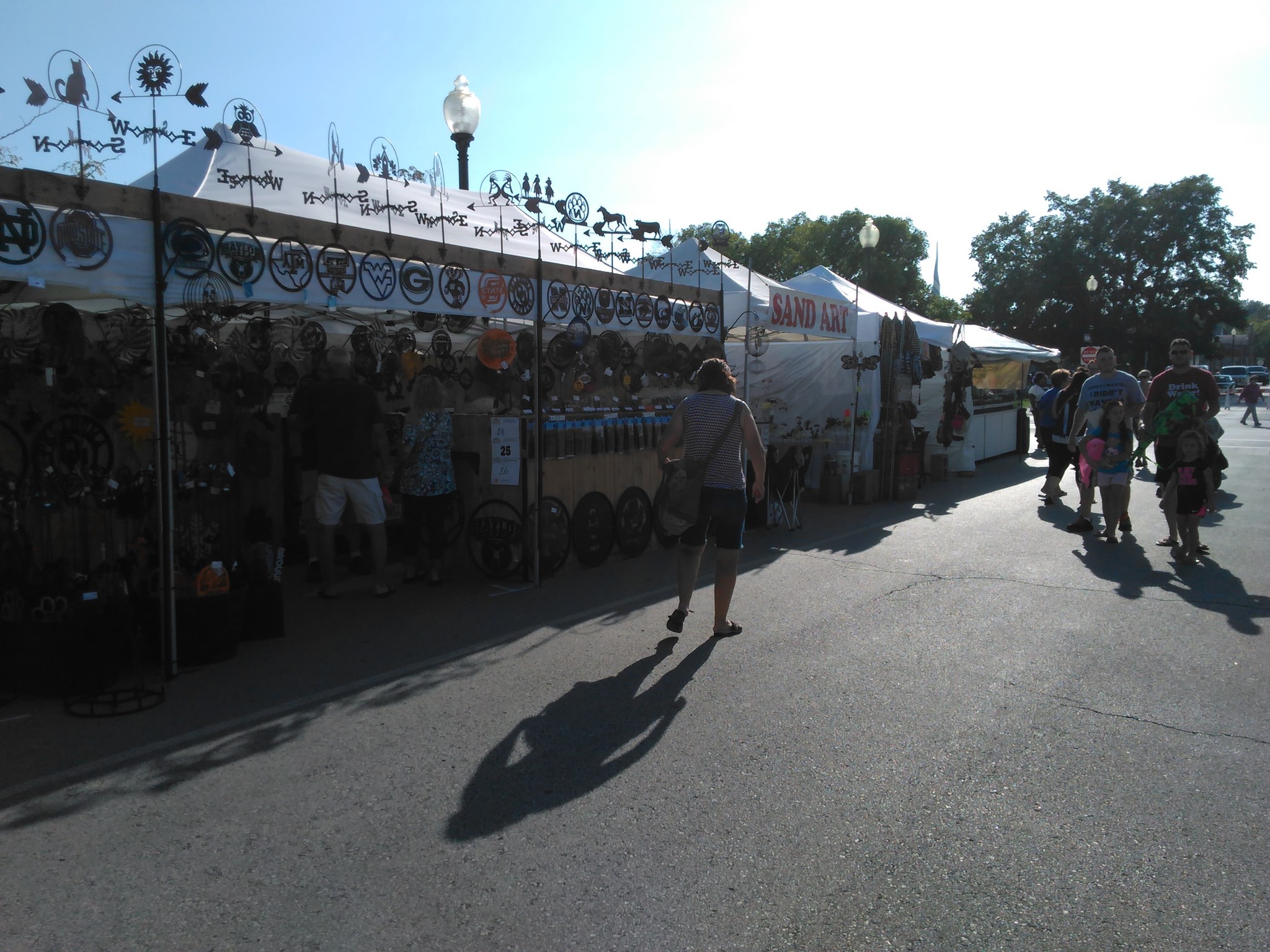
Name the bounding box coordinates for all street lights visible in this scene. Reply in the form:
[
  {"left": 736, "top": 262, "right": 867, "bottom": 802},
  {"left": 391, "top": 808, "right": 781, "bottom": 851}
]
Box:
[
  {"left": 1084, "top": 270, "right": 1099, "bottom": 334},
  {"left": 1230, "top": 328, "right": 1236, "bottom": 365},
  {"left": 857, "top": 215, "right": 880, "bottom": 291},
  {"left": 442, "top": 73, "right": 482, "bottom": 192}
]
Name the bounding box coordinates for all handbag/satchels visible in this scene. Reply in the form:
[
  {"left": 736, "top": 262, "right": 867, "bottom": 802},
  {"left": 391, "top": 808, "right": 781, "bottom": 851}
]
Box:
[{"left": 654, "top": 460, "right": 703, "bottom": 534}]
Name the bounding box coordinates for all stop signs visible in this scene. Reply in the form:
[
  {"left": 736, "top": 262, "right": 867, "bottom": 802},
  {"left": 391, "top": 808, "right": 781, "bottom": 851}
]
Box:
[{"left": 1080, "top": 345, "right": 1101, "bottom": 366}]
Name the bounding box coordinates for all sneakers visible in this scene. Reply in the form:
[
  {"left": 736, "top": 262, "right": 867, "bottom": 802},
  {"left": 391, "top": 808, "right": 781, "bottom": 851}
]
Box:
[
  {"left": 1118, "top": 515, "right": 1131, "bottom": 531},
  {"left": 1067, "top": 517, "right": 1093, "bottom": 531}
]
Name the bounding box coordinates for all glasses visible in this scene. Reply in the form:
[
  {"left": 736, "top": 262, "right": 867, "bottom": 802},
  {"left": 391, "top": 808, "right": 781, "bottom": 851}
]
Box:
[
  {"left": 1171, "top": 350, "right": 1190, "bottom": 354},
  {"left": 1138, "top": 376, "right": 1148, "bottom": 379}
]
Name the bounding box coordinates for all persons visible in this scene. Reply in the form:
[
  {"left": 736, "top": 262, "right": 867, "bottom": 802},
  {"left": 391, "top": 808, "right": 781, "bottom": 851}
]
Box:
[
  {"left": 1236, "top": 374, "right": 1265, "bottom": 426},
  {"left": 1027, "top": 359, "right": 1101, "bottom": 500},
  {"left": 1067, "top": 344, "right": 1146, "bottom": 534},
  {"left": 1077, "top": 399, "right": 1133, "bottom": 545},
  {"left": 656, "top": 357, "right": 766, "bottom": 637},
  {"left": 1136, "top": 369, "right": 1153, "bottom": 465},
  {"left": 286, "top": 345, "right": 399, "bottom": 599},
  {"left": 394, "top": 372, "right": 463, "bottom": 587},
  {"left": 1215, "top": 368, "right": 1220, "bottom": 375},
  {"left": 1159, "top": 430, "right": 1217, "bottom": 565},
  {"left": 1137, "top": 337, "right": 1220, "bottom": 552}
]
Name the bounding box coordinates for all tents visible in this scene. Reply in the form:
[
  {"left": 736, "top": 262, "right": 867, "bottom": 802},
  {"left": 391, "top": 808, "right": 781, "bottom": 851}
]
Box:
[
  {"left": 613, "top": 231, "right": 859, "bottom": 505},
  {"left": 939, "top": 319, "right": 1063, "bottom": 464},
  {"left": 781, "top": 261, "right": 957, "bottom": 477},
  {"left": 0, "top": 120, "right": 727, "bottom": 683}
]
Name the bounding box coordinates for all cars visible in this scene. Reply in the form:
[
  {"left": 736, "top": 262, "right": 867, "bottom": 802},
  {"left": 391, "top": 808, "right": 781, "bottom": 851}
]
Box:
[{"left": 1212, "top": 375, "right": 1236, "bottom": 395}]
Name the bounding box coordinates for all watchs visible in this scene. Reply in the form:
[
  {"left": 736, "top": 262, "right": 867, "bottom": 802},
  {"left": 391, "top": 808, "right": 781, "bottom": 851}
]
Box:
[{"left": 1136, "top": 433, "right": 1140, "bottom": 441}]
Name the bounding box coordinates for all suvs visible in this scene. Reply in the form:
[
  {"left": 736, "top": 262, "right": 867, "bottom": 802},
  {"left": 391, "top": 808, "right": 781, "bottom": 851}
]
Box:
[
  {"left": 1219, "top": 366, "right": 1251, "bottom": 389},
  {"left": 1247, "top": 365, "right": 1269, "bottom": 386}
]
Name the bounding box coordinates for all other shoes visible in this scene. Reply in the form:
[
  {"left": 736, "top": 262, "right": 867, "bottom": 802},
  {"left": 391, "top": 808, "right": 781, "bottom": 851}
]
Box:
[
  {"left": 1060, "top": 488, "right": 1067, "bottom": 497},
  {"left": 1254, "top": 422, "right": 1261, "bottom": 426},
  {"left": 404, "top": 568, "right": 445, "bottom": 586},
  {"left": 1173, "top": 544, "right": 1197, "bottom": 564},
  {"left": 1038, "top": 495, "right": 1059, "bottom": 505},
  {"left": 1135, "top": 460, "right": 1148, "bottom": 467},
  {"left": 350, "top": 555, "right": 366, "bottom": 574},
  {"left": 305, "top": 565, "right": 322, "bottom": 583},
  {"left": 1240, "top": 419, "right": 1247, "bottom": 425},
  {"left": 1079, "top": 501, "right": 1097, "bottom": 505},
  {"left": 1094, "top": 530, "right": 1117, "bottom": 543}
]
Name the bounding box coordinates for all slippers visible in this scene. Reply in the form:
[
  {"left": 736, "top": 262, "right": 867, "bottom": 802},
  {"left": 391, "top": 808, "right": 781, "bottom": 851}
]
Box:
[
  {"left": 375, "top": 584, "right": 396, "bottom": 598},
  {"left": 1197, "top": 542, "right": 1209, "bottom": 551},
  {"left": 667, "top": 610, "right": 688, "bottom": 633},
  {"left": 713, "top": 620, "right": 742, "bottom": 637},
  {"left": 318, "top": 585, "right": 340, "bottom": 599},
  {"left": 1155, "top": 537, "right": 1180, "bottom": 546}
]
